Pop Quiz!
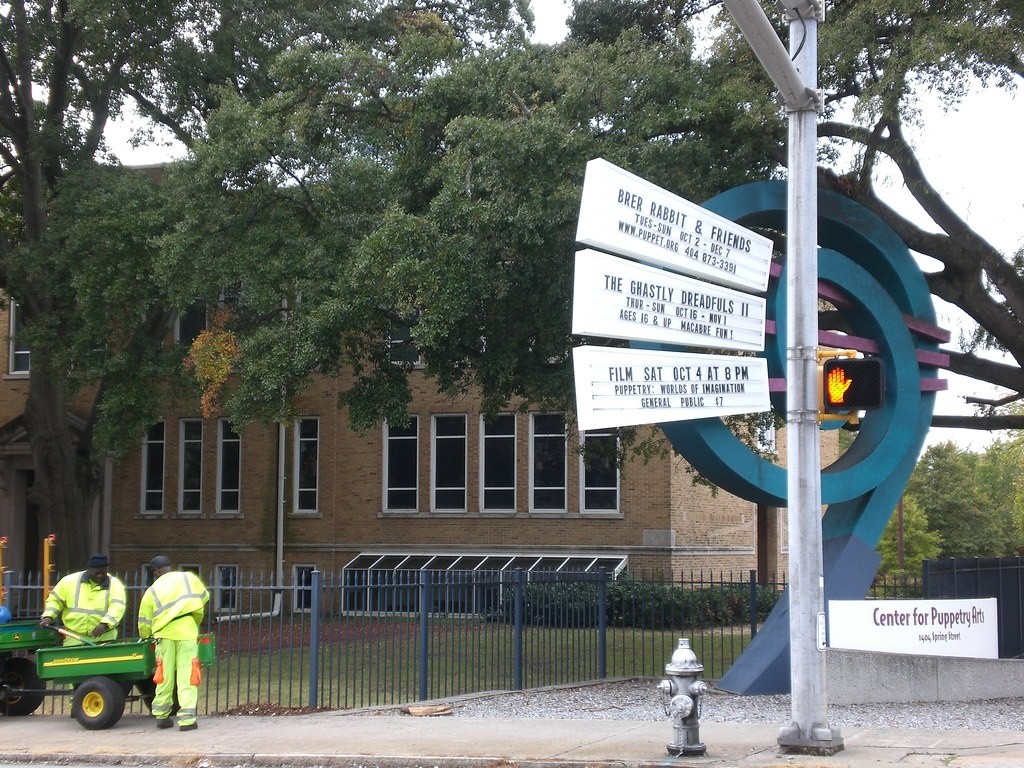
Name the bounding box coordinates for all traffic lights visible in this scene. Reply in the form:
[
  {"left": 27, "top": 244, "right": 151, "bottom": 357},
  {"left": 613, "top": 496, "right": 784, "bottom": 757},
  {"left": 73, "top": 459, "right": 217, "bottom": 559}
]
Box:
[{"left": 822, "top": 356, "right": 887, "bottom": 412}]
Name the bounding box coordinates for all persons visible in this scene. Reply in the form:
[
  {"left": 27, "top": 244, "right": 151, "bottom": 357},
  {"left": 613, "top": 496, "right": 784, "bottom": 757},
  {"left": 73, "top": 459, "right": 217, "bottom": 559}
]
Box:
[
  {"left": 40, "top": 553, "right": 126, "bottom": 717},
  {"left": 138, "top": 556, "right": 210, "bottom": 731}
]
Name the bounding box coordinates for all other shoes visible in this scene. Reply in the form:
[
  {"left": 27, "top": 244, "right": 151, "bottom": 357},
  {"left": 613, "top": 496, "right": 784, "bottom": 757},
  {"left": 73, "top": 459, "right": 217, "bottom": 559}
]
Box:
[
  {"left": 156, "top": 717, "right": 174, "bottom": 728},
  {"left": 178, "top": 721, "right": 198, "bottom": 731}
]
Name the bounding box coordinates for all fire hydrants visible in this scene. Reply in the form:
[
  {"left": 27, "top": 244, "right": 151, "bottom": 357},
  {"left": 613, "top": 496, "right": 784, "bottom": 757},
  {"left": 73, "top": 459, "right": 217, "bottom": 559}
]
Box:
[{"left": 655, "top": 636, "right": 711, "bottom": 758}]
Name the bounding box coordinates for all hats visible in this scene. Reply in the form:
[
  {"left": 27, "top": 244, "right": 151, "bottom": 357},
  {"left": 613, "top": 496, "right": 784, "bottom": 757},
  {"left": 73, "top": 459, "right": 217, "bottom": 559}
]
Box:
[
  {"left": 86, "top": 553, "right": 109, "bottom": 567},
  {"left": 150, "top": 554, "right": 171, "bottom": 569}
]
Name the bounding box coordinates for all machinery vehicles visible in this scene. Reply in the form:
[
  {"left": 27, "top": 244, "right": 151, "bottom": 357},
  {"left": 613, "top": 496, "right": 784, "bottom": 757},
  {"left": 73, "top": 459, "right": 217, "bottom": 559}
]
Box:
[{"left": 0, "top": 533, "right": 219, "bottom": 731}]
